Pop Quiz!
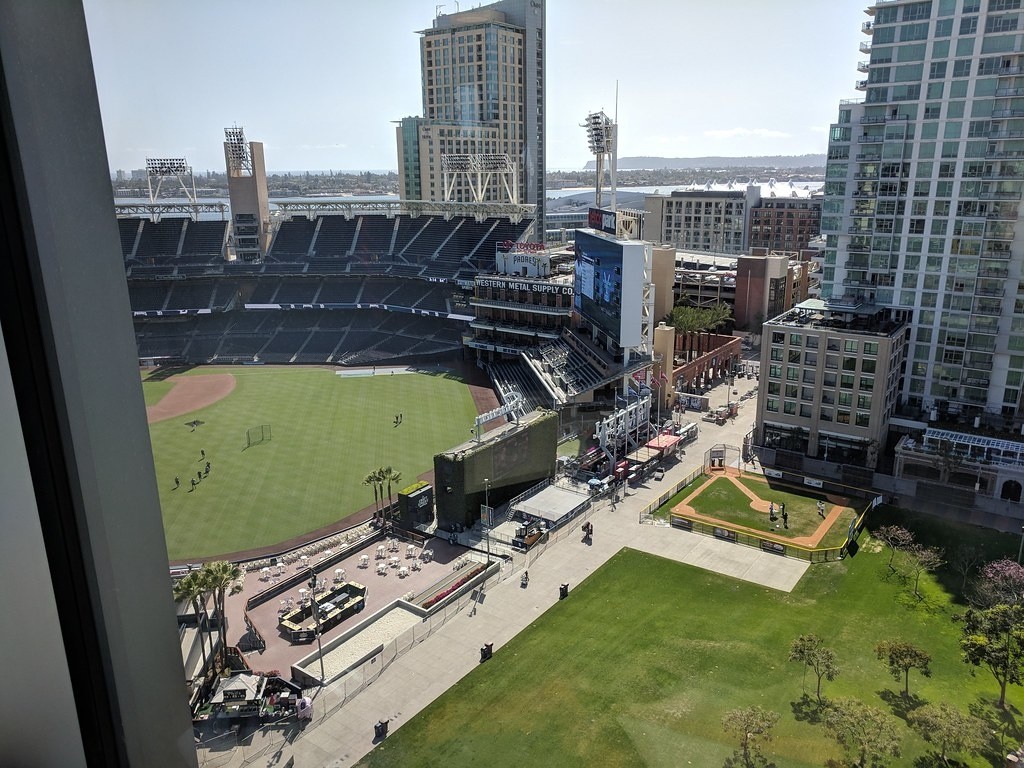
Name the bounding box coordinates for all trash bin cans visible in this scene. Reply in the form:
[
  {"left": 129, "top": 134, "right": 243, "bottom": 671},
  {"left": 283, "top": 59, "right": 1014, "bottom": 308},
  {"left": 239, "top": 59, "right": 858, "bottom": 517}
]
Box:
[
  {"left": 479, "top": 641, "right": 493, "bottom": 664},
  {"left": 374, "top": 717, "right": 389, "bottom": 737},
  {"left": 559, "top": 583, "right": 569, "bottom": 599}
]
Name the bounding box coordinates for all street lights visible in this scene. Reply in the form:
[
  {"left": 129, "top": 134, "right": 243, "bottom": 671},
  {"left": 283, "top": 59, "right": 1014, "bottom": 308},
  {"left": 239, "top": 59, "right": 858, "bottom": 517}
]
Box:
[
  {"left": 726, "top": 352, "right": 735, "bottom": 406},
  {"left": 482, "top": 478, "right": 495, "bottom": 566},
  {"left": 309, "top": 567, "right": 324, "bottom": 682},
  {"left": 675, "top": 392, "right": 686, "bottom": 441}
]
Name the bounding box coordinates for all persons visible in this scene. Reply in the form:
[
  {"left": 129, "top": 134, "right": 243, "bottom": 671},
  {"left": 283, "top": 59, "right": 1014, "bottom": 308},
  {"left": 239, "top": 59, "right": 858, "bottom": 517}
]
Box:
[
  {"left": 769, "top": 502, "right": 776, "bottom": 520},
  {"left": 201, "top": 448, "right": 205, "bottom": 458},
  {"left": 175, "top": 476, "right": 180, "bottom": 489},
  {"left": 781, "top": 502, "right": 785, "bottom": 515},
  {"left": 191, "top": 462, "right": 211, "bottom": 490},
  {"left": 395, "top": 411, "right": 403, "bottom": 424},
  {"left": 817, "top": 500, "right": 822, "bottom": 515},
  {"left": 192, "top": 419, "right": 198, "bottom": 431},
  {"left": 821, "top": 502, "right": 825, "bottom": 517},
  {"left": 783, "top": 513, "right": 788, "bottom": 525}
]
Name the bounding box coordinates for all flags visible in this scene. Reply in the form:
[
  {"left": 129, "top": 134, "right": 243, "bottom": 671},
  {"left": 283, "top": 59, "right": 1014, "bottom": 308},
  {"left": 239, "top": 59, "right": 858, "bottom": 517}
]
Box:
[
  {"left": 628, "top": 385, "right": 641, "bottom": 397},
  {"left": 660, "top": 370, "right": 669, "bottom": 383},
  {"left": 640, "top": 381, "right": 655, "bottom": 392},
  {"left": 615, "top": 391, "right": 628, "bottom": 401},
  {"left": 650, "top": 375, "right": 662, "bottom": 388}
]
